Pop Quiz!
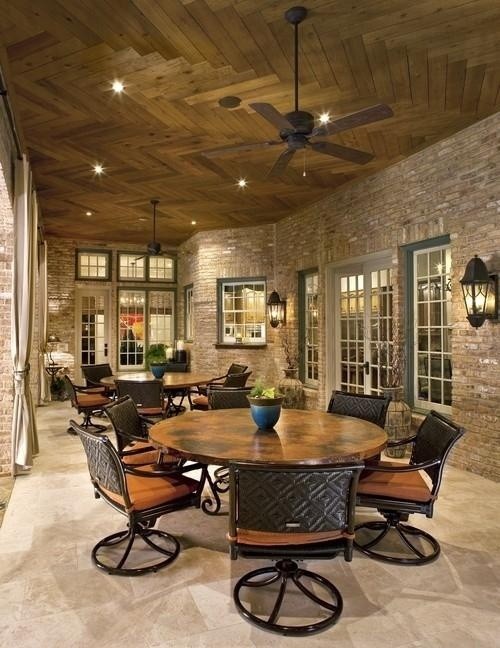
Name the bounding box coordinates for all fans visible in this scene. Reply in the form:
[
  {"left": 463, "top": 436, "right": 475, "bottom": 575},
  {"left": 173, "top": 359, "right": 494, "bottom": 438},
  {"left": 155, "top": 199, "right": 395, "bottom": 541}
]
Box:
[
  {"left": 204, "top": 7, "right": 394, "bottom": 181},
  {"left": 132, "top": 200, "right": 181, "bottom": 261}
]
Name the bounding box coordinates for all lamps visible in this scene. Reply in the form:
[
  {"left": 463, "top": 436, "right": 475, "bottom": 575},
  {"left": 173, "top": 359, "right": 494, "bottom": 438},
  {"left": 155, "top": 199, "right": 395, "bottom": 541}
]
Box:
[
  {"left": 459, "top": 254, "right": 499, "bottom": 331},
  {"left": 266, "top": 288, "right": 286, "bottom": 328}
]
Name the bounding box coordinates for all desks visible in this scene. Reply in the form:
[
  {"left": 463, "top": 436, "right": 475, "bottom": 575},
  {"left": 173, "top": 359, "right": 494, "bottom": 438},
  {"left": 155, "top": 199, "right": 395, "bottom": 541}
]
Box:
[{"left": 149, "top": 407, "right": 388, "bottom": 516}]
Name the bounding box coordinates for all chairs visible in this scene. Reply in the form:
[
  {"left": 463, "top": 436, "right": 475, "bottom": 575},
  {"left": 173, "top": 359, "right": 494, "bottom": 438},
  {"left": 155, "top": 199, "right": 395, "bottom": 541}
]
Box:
[
  {"left": 327, "top": 389, "right": 393, "bottom": 430},
  {"left": 164, "top": 363, "right": 187, "bottom": 416},
  {"left": 115, "top": 380, "right": 171, "bottom": 426},
  {"left": 81, "top": 363, "right": 117, "bottom": 418},
  {"left": 224, "top": 458, "right": 364, "bottom": 639},
  {"left": 187, "top": 371, "right": 252, "bottom": 410},
  {"left": 207, "top": 363, "right": 247, "bottom": 387},
  {"left": 352, "top": 408, "right": 466, "bottom": 567},
  {"left": 206, "top": 385, "right": 251, "bottom": 410},
  {"left": 68, "top": 420, "right": 220, "bottom": 576},
  {"left": 102, "top": 393, "right": 187, "bottom": 467},
  {"left": 63, "top": 374, "right": 114, "bottom": 434}
]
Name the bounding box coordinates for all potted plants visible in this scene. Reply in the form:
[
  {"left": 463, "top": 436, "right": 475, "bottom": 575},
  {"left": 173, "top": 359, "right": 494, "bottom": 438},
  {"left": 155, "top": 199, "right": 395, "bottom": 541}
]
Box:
[
  {"left": 379, "top": 353, "right": 411, "bottom": 458},
  {"left": 246, "top": 378, "right": 284, "bottom": 433},
  {"left": 278, "top": 331, "right": 303, "bottom": 409},
  {"left": 145, "top": 342, "right": 167, "bottom": 379}
]
